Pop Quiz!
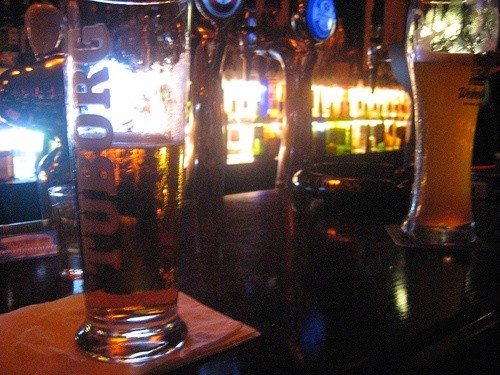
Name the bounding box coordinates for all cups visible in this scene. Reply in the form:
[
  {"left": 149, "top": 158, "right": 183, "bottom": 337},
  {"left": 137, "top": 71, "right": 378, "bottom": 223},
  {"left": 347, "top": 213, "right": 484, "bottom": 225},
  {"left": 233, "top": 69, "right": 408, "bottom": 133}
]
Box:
[
  {"left": 64, "top": 0, "right": 190, "bottom": 365},
  {"left": 399, "top": 0, "right": 500, "bottom": 250},
  {"left": 48, "top": 184, "right": 83, "bottom": 280}
]
